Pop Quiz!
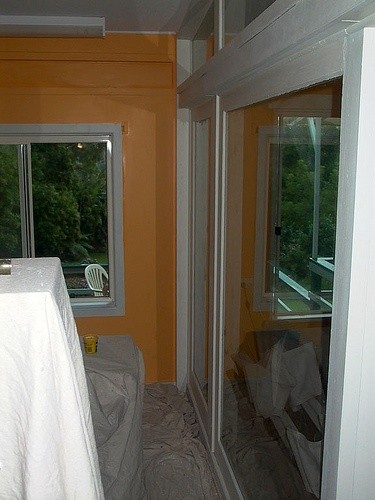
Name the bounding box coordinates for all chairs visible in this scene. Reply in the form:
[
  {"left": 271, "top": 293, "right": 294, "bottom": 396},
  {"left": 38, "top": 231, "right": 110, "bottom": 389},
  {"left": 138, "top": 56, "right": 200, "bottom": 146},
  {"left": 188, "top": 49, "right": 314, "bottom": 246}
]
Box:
[{"left": 84, "top": 264, "right": 108, "bottom": 297}]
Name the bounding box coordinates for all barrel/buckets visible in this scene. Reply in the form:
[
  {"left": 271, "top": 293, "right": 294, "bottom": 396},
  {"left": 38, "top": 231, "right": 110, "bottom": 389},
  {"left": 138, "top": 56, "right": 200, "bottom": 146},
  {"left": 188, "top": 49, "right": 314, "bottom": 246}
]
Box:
[{"left": 83, "top": 334, "right": 97, "bottom": 354}]
